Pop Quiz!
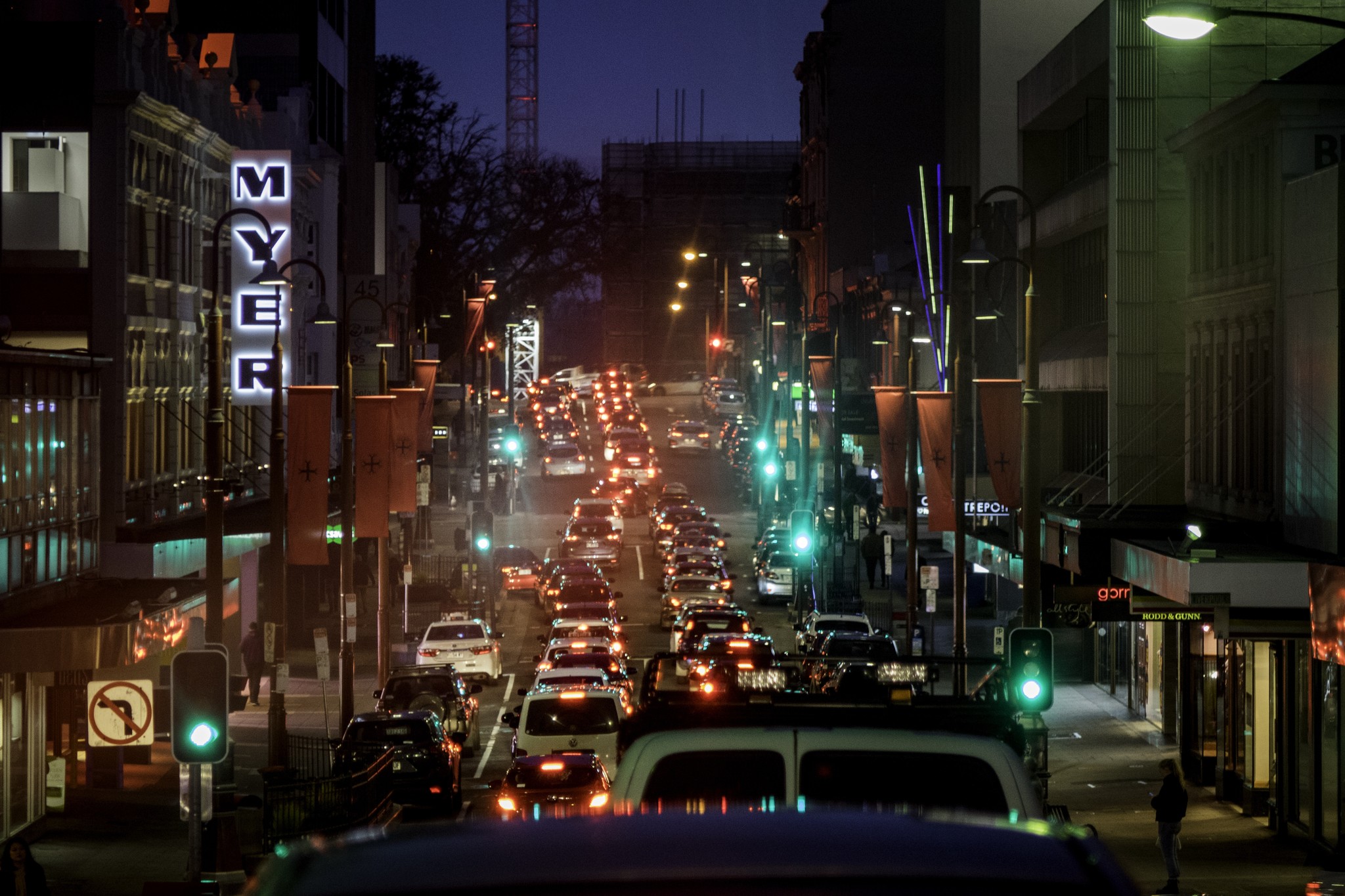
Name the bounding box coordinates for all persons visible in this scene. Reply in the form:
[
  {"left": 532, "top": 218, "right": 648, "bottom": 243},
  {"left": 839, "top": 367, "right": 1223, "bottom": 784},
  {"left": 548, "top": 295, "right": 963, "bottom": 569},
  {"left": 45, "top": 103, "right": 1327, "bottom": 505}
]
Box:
[
  {"left": 353, "top": 555, "right": 375, "bottom": 617},
  {"left": 239, "top": 621, "right": 265, "bottom": 706},
  {"left": 878, "top": 530, "right": 895, "bottom": 590},
  {"left": 859, "top": 522, "right": 882, "bottom": 590},
  {"left": 388, "top": 548, "right": 404, "bottom": 611},
  {"left": 323, "top": 562, "right": 340, "bottom": 615},
  {"left": 0, "top": 837, "right": 46, "bottom": 896},
  {"left": 904, "top": 554, "right": 927, "bottom": 611},
  {"left": 1150, "top": 758, "right": 1189, "bottom": 896}
]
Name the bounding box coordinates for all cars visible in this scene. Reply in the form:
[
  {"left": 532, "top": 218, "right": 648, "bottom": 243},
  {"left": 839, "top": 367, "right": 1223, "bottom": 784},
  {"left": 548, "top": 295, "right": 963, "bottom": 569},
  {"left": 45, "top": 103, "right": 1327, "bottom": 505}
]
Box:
[
  {"left": 494, "top": 361, "right": 1153, "bottom": 823},
  {"left": 372, "top": 663, "right": 483, "bottom": 758},
  {"left": 330, "top": 709, "right": 468, "bottom": 818},
  {"left": 494, "top": 546, "right": 542, "bottom": 593},
  {"left": 413, "top": 619, "right": 505, "bottom": 686}
]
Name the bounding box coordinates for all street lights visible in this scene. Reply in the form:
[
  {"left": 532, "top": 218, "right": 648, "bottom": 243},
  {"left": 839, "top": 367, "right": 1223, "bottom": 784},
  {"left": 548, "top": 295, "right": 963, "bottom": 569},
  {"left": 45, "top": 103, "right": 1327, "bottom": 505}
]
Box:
[
  {"left": 962, "top": 183, "right": 1043, "bottom": 637},
  {"left": 871, "top": 299, "right": 950, "bottom": 663},
  {"left": 200, "top": 209, "right": 289, "bottom": 669},
  {"left": 669, "top": 237, "right": 848, "bottom": 560},
  {"left": 341, "top": 245, "right": 522, "bottom": 752},
  {"left": 269, "top": 258, "right": 339, "bottom": 769},
  {"left": 805, "top": 293, "right": 841, "bottom": 589}
]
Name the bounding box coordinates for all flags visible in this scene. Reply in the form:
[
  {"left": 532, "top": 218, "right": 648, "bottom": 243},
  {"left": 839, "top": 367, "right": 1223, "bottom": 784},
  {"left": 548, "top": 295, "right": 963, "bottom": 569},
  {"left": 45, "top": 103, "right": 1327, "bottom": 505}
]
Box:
[
  {"left": 413, "top": 359, "right": 437, "bottom": 452},
  {"left": 478, "top": 280, "right": 494, "bottom": 309},
  {"left": 465, "top": 298, "right": 485, "bottom": 354},
  {"left": 915, "top": 391, "right": 957, "bottom": 532},
  {"left": 874, "top": 386, "right": 907, "bottom": 507},
  {"left": 390, "top": 388, "right": 420, "bottom": 512},
  {"left": 809, "top": 355, "right": 835, "bottom": 447},
  {"left": 978, "top": 379, "right": 1024, "bottom": 532},
  {"left": 286, "top": 385, "right": 332, "bottom": 566},
  {"left": 355, "top": 395, "right": 391, "bottom": 539}
]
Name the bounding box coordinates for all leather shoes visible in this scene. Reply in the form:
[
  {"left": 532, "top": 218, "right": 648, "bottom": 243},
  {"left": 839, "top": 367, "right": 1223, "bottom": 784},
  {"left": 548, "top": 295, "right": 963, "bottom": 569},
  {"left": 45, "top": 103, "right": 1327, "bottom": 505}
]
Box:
[{"left": 1155, "top": 880, "right": 1180, "bottom": 895}]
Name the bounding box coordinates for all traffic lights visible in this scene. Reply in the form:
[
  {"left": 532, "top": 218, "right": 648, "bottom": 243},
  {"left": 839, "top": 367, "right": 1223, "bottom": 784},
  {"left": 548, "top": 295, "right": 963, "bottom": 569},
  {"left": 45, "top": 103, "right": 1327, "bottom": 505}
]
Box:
[
  {"left": 788, "top": 499, "right": 817, "bottom": 567},
  {"left": 468, "top": 509, "right": 494, "bottom": 567},
  {"left": 500, "top": 423, "right": 529, "bottom": 469},
  {"left": 752, "top": 431, "right": 784, "bottom": 488},
  {"left": 1006, "top": 627, "right": 1055, "bottom": 711},
  {"left": 170, "top": 642, "right": 229, "bottom": 765}
]
